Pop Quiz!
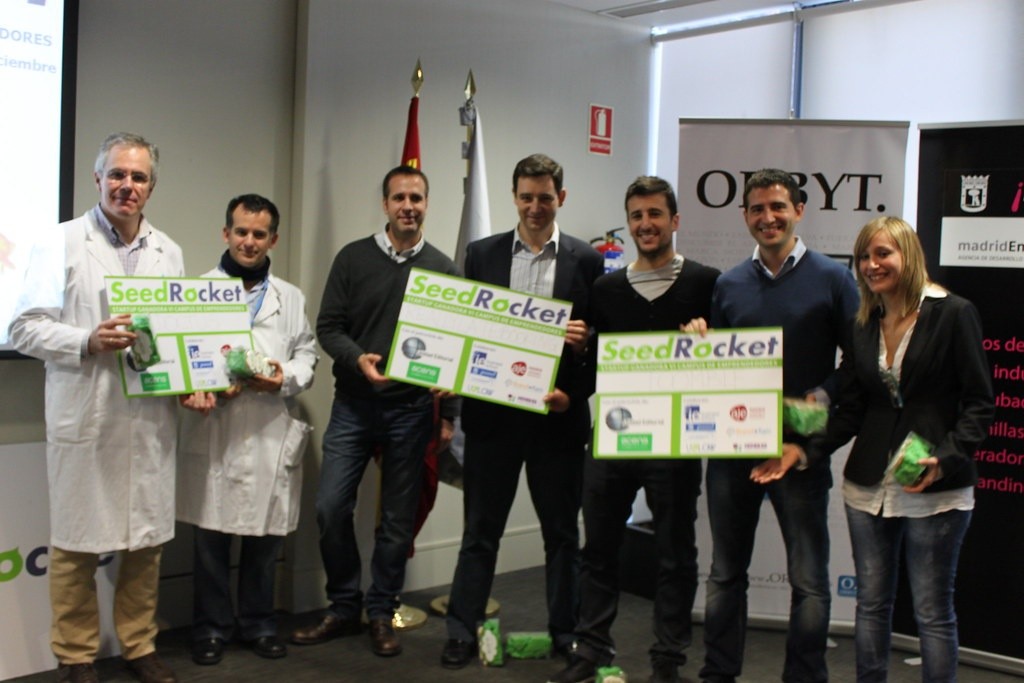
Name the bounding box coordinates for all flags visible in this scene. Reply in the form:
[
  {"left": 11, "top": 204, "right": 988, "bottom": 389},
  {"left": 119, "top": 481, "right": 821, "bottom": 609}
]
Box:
[
  {"left": 373, "top": 97, "right": 443, "bottom": 556},
  {"left": 440, "top": 110, "right": 492, "bottom": 490}
]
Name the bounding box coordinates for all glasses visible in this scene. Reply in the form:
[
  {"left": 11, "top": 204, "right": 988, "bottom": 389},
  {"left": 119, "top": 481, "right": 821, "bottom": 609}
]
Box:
[{"left": 99, "top": 170, "right": 151, "bottom": 185}]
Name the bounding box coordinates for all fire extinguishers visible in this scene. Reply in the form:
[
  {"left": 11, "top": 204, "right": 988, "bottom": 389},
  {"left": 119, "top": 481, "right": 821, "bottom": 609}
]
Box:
[{"left": 588, "top": 227, "right": 625, "bottom": 275}]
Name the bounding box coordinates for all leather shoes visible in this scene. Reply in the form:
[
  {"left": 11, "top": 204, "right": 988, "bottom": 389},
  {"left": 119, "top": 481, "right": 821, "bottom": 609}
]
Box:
[
  {"left": 368, "top": 619, "right": 402, "bottom": 654},
  {"left": 289, "top": 612, "right": 362, "bottom": 644},
  {"left": 441, "top": 639, "right": 476, "bottom": 669},
  {"left": 192, "top": 637, "right": 224, "bottom": 664},
  {"left": 560, "top": 639, "right": 582, "bottom": 665},
  {"left": 58, "top": 661, "right": 98, "bottom": 683},
  {"left": 546, "top": 659, "right": 611, "bottom": 683},
  {"left": 645, "top": 669, "right": 680, "bottom": 683},
  {"left": 125, "top": 652, "right": 175, "bottom": 683},
  {"left": 249, "top": 636, "right": 287, "bottom": 657}
]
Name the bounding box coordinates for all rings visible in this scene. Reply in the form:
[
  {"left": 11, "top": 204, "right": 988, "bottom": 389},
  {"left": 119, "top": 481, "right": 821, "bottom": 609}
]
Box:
[{"left": 109, "top": 338, "right": 113, "bottom": 346}]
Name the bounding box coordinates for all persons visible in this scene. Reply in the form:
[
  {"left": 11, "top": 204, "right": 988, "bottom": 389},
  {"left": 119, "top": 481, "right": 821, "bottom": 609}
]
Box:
[
  {"left": 682, "top": 167, "right": 858, "bottom": 683},
  {"left": 548, "top": 174, "right": 722, "bottom": 683},
  {"left": 7, "top": 133, "right": 208, "bottom": 683},
  {"left": 748, "top": 216, "right": 994, "bottom": 683},
  {"left": 441, "top": 155, "right": 605, "bottom": 672},
  {"left": 292, "top": 165, "right": 462, "bottom": 656},
  {"left": 173, "top": 194, "right": 318, "bottom": 663}
]
